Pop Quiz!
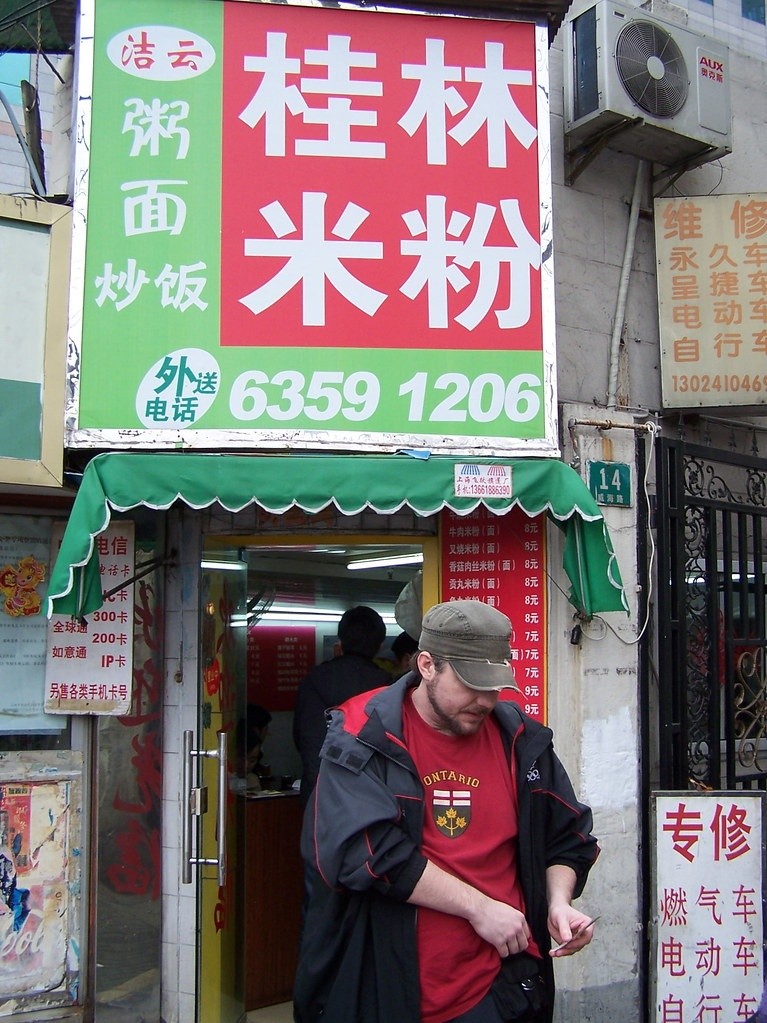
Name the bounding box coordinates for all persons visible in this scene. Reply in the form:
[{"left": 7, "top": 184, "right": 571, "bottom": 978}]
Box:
[
  {"left": 390, "top": 631, "right": 419, "bottom": 682},
  {"left": 290, "top": 606, "right": 393, "bottom": 808},
  {"left": 236, "top": 701, "right": 272, "bottom": 779},
  {"left": 291, "top": 600, "right": 601, "bottom": 1023}
]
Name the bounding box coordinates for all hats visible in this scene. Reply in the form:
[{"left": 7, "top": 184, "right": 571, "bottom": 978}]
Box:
[{"left": 417, "top": 599, "right": 527, "bottom": 698}]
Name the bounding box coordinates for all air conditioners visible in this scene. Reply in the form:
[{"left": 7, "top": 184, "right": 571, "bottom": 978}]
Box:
[{"left": 563, "top": 0, "right": 730, "bottom": 167}]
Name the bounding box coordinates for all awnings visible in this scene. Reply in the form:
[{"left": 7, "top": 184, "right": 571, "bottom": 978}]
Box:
[{"left": 41, "top": 453, "right": 628, "bottom": 618}]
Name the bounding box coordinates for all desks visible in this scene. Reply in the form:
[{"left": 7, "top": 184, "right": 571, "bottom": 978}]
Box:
[{"left": 236, "top": 789, "right": 306, "bottom": 1013}]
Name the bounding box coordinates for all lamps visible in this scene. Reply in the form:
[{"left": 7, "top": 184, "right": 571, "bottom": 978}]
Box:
[
  {"left": 343, "top": 550, "right": 423, "bottom": 571},
  {"left": 200, "top": 550, "right": 248, "bottom": 571},
  {"left": 230, "top": 605, "right": 400, "bottom": 627}
]
